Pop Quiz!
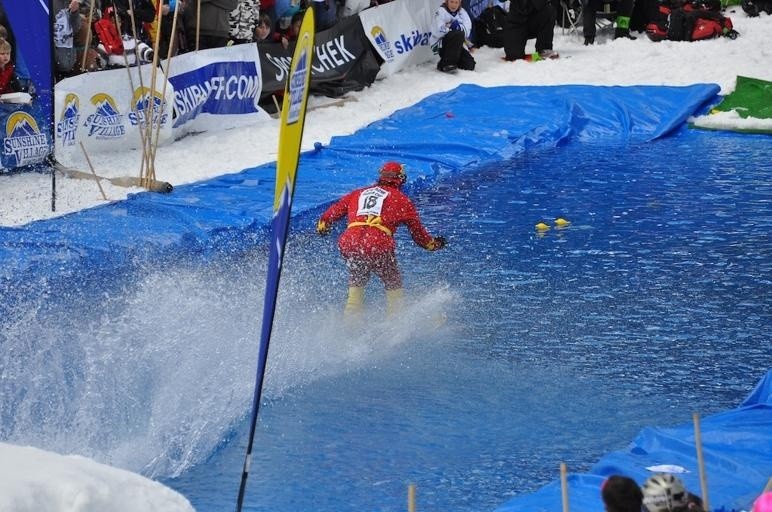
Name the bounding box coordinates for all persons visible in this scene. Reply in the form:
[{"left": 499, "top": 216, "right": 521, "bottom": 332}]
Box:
[
  {"left": 430, "top": 0, "right": 559, "bottom": 73},
  {"left": 742, "top": 0, "right": 772, "bottom": 17},
  {"left": 54, "top": 1, "right": 386, "bottom": 81},
  {"left": 582, "top": 0, "right": 721, "bottom": 46},
  {"left": 316, "top": 160, "right": 447, "bottom": 327},
  {"left": 0, "top": 25, "right": 30, "bottom": 91}
]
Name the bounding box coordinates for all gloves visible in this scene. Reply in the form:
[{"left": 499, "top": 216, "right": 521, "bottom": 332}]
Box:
[
  {"left": 316, "top": 227, "right": 333, "bottom": 238},
  {"left": 432, "top": 234, "right": 450, "bottom": 250}
]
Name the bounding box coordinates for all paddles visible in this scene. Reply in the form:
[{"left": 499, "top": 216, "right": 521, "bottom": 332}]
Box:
[{"left": 238, "top": 6, "right": 314, "bottom": 512}]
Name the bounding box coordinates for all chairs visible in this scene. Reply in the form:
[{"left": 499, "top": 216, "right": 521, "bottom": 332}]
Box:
[{"left": 560, "top": 0, "right": 617, "bottom": 41}]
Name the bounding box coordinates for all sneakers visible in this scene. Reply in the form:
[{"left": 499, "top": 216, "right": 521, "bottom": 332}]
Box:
[
  {"left": 438, "top": 63, "right": 458, "bottom": 74},
  {"left": 538, "top": 49, "right": 560, "bottom": 60}
]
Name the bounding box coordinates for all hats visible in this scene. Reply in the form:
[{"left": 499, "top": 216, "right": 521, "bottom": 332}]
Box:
[{"left": 377, "top": 161, "right": 407, "bottom": 187}]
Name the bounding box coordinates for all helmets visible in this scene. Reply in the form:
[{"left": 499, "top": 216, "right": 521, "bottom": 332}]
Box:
[{"left": 639, "top": 473, "right": 691, "bottom": 512}]
